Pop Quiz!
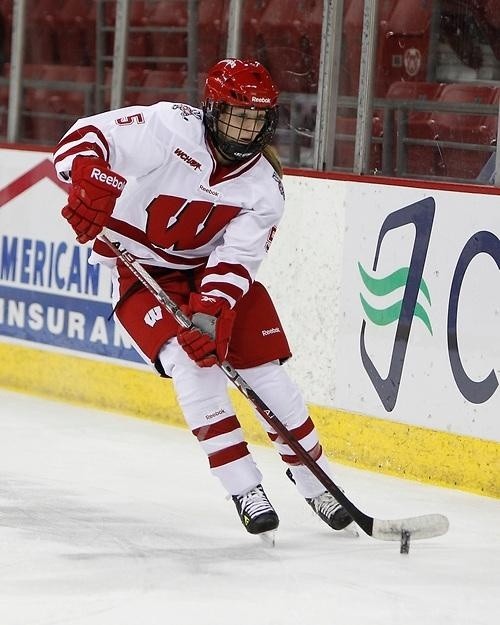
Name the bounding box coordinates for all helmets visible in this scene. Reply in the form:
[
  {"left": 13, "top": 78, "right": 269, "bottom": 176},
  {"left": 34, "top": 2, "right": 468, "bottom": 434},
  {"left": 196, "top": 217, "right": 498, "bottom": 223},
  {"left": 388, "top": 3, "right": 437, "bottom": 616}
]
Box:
[{"left": 201, "top": 57, "right": 280, "bottom": 109}]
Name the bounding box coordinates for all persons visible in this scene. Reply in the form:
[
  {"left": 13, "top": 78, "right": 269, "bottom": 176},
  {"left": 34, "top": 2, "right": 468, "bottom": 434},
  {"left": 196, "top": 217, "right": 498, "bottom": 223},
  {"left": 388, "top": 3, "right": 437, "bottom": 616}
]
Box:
[{"left": 54, "top": 59, "right": 357, "bottom": 534}]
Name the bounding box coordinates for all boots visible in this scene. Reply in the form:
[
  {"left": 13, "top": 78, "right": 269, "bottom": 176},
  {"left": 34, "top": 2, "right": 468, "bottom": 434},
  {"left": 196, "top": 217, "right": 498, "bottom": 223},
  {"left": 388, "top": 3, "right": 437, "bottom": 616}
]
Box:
[
  {"left": 231, "top": 484, "right": 279, "bottom": 535},
  {"left": 286, "top": 466, "right": 354, "bottom": 531}
]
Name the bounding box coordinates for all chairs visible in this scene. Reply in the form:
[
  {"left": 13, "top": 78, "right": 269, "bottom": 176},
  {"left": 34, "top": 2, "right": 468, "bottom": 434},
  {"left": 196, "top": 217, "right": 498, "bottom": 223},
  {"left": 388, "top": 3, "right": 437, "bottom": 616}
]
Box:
[{"left": 0, "top": 2, "right": 500, "bottom": 179}]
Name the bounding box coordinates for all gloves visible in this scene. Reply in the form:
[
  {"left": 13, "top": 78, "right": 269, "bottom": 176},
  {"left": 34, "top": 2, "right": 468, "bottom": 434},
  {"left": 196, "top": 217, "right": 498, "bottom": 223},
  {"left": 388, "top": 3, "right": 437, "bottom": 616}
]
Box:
[
  {"left": 176, "top": 290, "right": 240, "bottom": 368},
  {"left": 61, "top": 156, "right": 127, "bottom": 244}
]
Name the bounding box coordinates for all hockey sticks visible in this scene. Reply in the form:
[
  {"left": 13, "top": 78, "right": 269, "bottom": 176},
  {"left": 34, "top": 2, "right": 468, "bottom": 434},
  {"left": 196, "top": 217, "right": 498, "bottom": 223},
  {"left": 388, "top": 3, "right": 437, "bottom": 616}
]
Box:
[{"left": 100, "top": 234, "right": 449, "bottom": 541}]
